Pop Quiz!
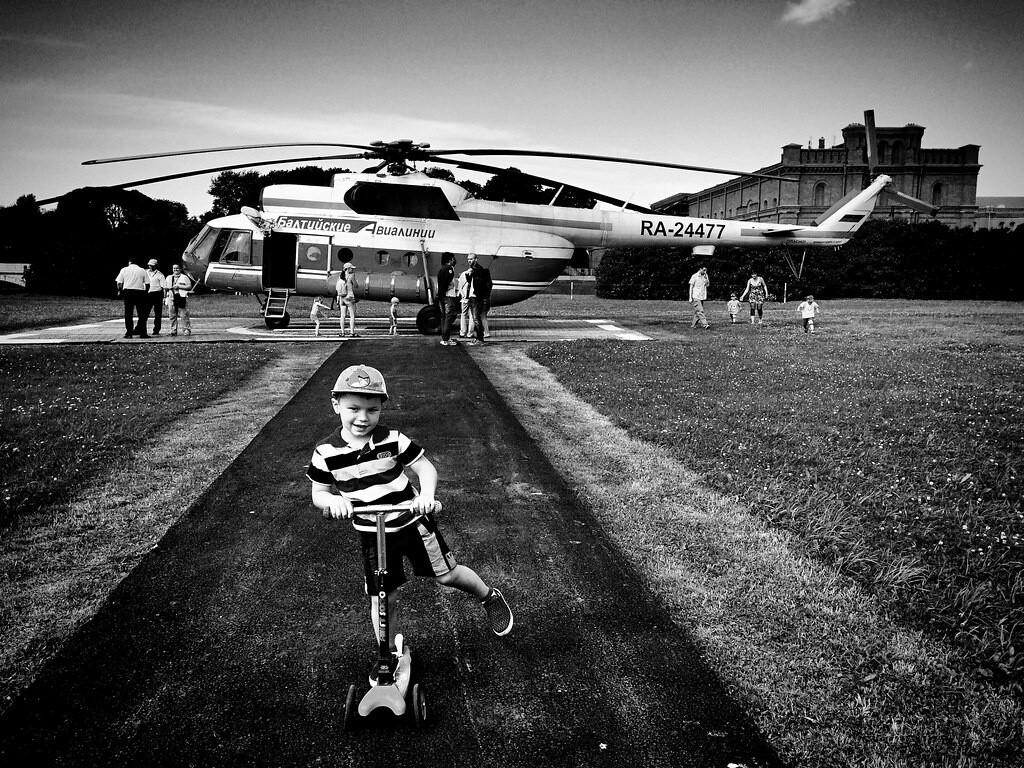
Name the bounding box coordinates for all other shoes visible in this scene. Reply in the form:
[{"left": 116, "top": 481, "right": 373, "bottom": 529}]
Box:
[
  {"left": 440, "top": 338, "right": 457, "bottom": 346},
  {"left": 339, "top": 333, "right": 345, "bottom": 337},
  {"left": 467, "top": 339, "right": 484, "bottom": 347},
  {"left": 350, "top": 333, "right": 361, "bottom": 337}
]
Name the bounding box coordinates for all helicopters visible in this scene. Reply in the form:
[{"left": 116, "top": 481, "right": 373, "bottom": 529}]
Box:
[{"left": 32, "top": 110, "right": 943, "bottom": 335}]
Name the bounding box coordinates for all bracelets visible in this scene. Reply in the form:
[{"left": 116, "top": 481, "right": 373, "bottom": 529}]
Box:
[{"left": 163, "top": 297, "right": 166, "bottom": 298}]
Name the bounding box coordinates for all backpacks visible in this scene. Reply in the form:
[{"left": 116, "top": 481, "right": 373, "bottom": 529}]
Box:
[{"left": 336, "top": 274, "right": 350, "bottom": 296}]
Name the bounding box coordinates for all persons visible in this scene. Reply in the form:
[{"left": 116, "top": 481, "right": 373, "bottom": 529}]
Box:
[
  {"left": 388, "top": 297, "right": 399, "bottom": 335},
  {"left": 163, "top": 263, "right": 192, "bottom": 336},
  {"left": 310, "top": 296, "right": 331, "bottom": 336},
  {"left": 306, "top": 363, "right": 514, "bottom": 687},
  {"left": 689, "top": 264, "right": 710, "bottom": 329},
  {"left": 133, "top": 259, "right": 167, "bottom": 336},
  {"left": 740, "top": 270, "right": 768, "bottom": 324},
  {"left": 336, "top": 263, "right": 361, "bottom": 337},
  {"left": 435, "top": 252, "right": 493, "bottom": 345},
  {"left": 115, "top": 257, "right": 152, "bottom": 338},
  {"left": 727, "top": 293, "right": 741, "bottom": 325},
  {"left": 797, "top": 295, "right": 820, "bottom": 334}
]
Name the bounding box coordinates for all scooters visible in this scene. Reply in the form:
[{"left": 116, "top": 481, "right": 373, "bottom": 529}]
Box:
[{"left": 324, "top": 500, "right": 442, "bottom": 732}]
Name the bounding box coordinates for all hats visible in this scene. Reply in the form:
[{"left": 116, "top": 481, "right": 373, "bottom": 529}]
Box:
[
  {"left": 343, "top": 262, "right": 356, "bottom": 269},
  {"left": 331, "top": 364, "right": 388, "bottom": 399}
]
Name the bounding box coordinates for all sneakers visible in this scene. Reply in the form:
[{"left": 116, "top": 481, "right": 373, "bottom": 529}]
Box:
[
  {"left": 482, "top": 588, "right": 513, "bottom": 636},
  {"left": 369, "top": 653, "right": 400, "bottom": 688}
]
